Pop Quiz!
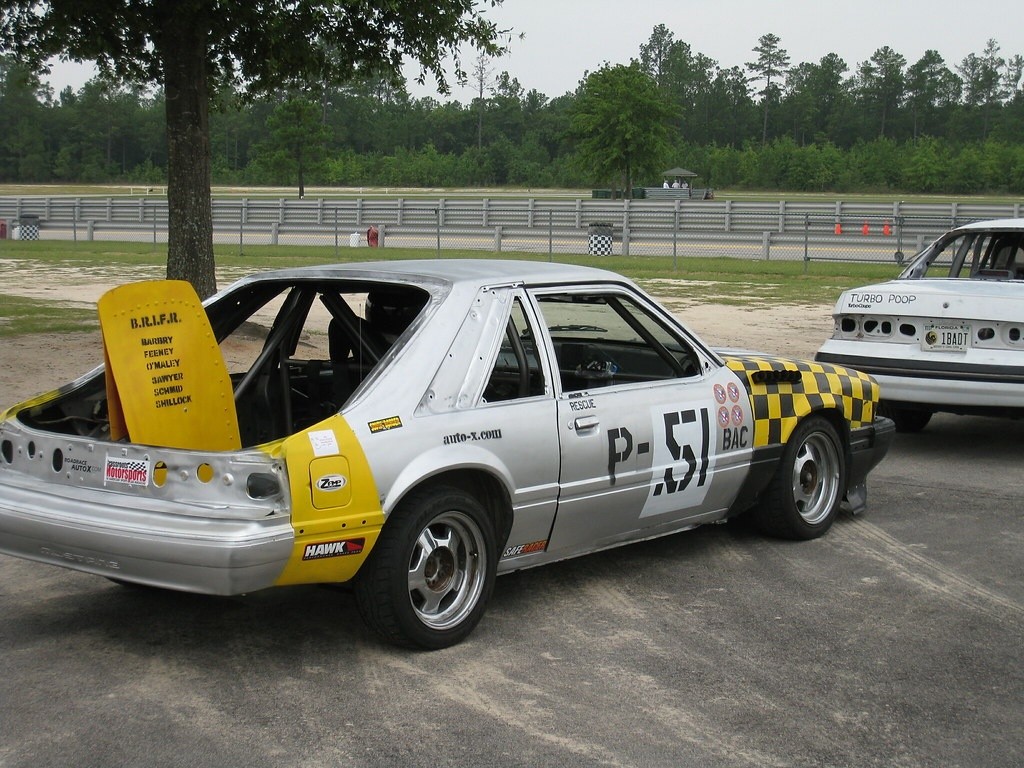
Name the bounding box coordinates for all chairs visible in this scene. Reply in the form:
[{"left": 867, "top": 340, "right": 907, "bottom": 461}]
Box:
[{"left": 365, "top": 290, "right": 424, "bottom": 336}]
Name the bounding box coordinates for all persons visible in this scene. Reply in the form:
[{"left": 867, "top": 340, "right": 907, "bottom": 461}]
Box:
[
  {"left": 664, "top": 180, "right": 669, "bottom": 188},
  {"left": 681, "top": 181, "right": 688, "bottom": 188},
  {"left": 672, "top": 180, "right": 679, "bottom": 188}
]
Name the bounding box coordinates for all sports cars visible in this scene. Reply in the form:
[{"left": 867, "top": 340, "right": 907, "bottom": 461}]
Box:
[{"left": 1, "top": 262, "right": 898, "bottom": 653}]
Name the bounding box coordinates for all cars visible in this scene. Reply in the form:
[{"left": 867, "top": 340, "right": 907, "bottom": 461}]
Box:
[{"left": 814, "top": 219, "right": 1024, "bottom": 434}]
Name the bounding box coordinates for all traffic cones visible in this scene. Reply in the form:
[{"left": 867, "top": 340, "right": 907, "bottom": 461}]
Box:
[
  {"left": 884, "top": 220, "right": 892, "bottom": 235},
  {"left": 862, "top": 220, "right": 869, "bottom": 235},
  {"left": 835, "top": 222, "right": 841, "bottom": 234}
]
